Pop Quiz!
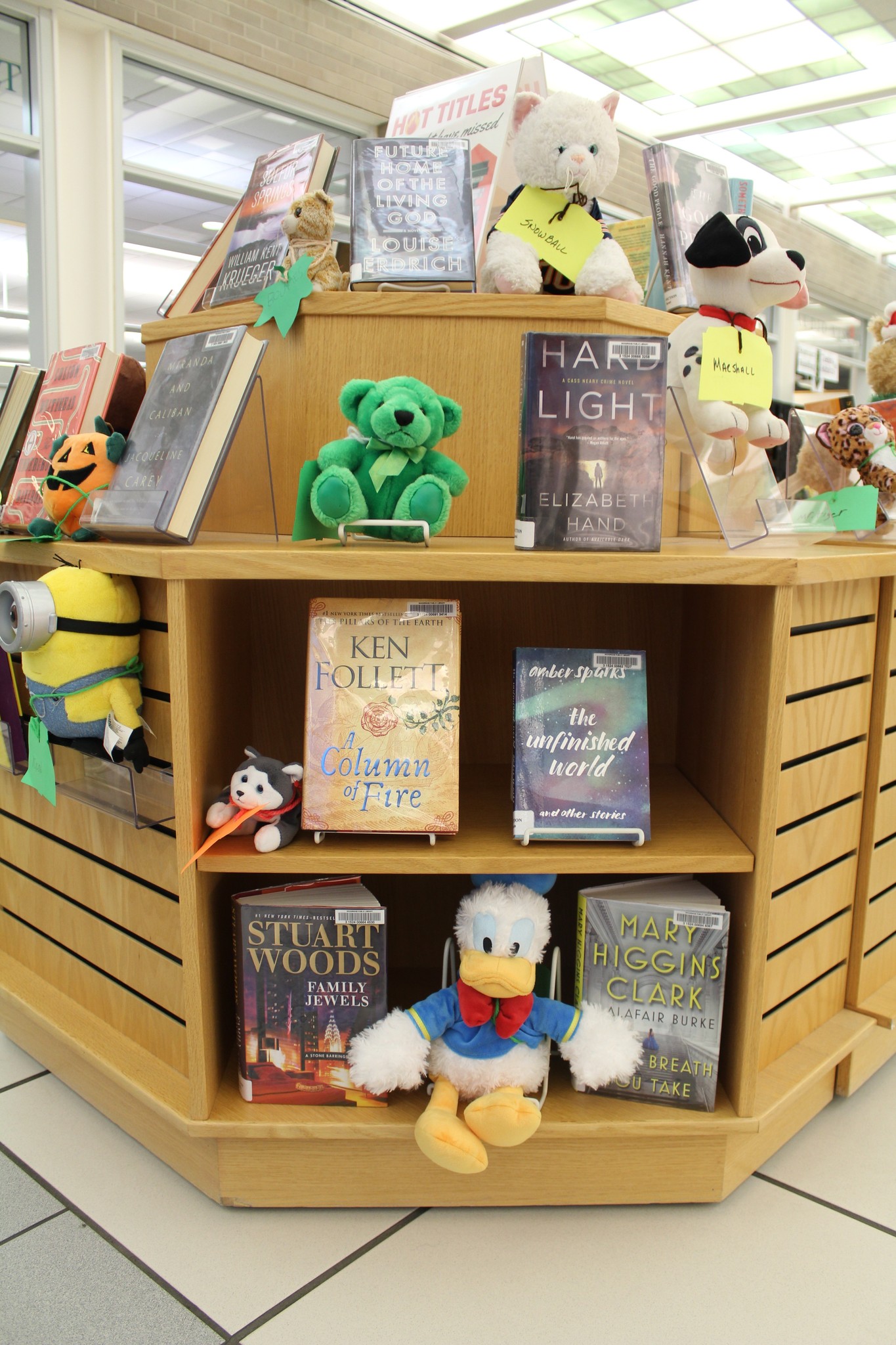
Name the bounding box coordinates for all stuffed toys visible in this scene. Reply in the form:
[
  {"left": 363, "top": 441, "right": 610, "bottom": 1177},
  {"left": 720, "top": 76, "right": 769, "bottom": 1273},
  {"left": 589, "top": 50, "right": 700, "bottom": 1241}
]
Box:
[
  {"left": 278, "top": 189, "right": 350, "bottom": 294},
  {"left": 309, "top": 375, "right": 469, "bottom": 541},
  {"left": 30, "top": 417, "right": 125, "bottom": 544},
  {"left": 0, "top": 128, "right": 860, "bottom": 1110},
  {"left": 0, "top": 553, "right": 150, "bottom": 774},
  {"left": 480, "top": 90, "right": 645, "bottom": 304},
  {"left": 790, "top": 300, "right": 896, "bottom": 533},
  {"left": 350, "top": 875, "right": 651, "bottom": 1174},
  {"left": 205, "top": 746, "right": 302, "bottom": 854},
  {"left": 664, "top": 212, "right": 809, "bottom": 477}
]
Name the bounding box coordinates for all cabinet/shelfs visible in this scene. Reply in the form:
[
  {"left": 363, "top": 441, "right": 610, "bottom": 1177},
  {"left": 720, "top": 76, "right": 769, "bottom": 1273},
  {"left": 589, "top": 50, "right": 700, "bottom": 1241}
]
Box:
[{"left": 0, "top": 294, "right": 896, "bottom": 1206}]
